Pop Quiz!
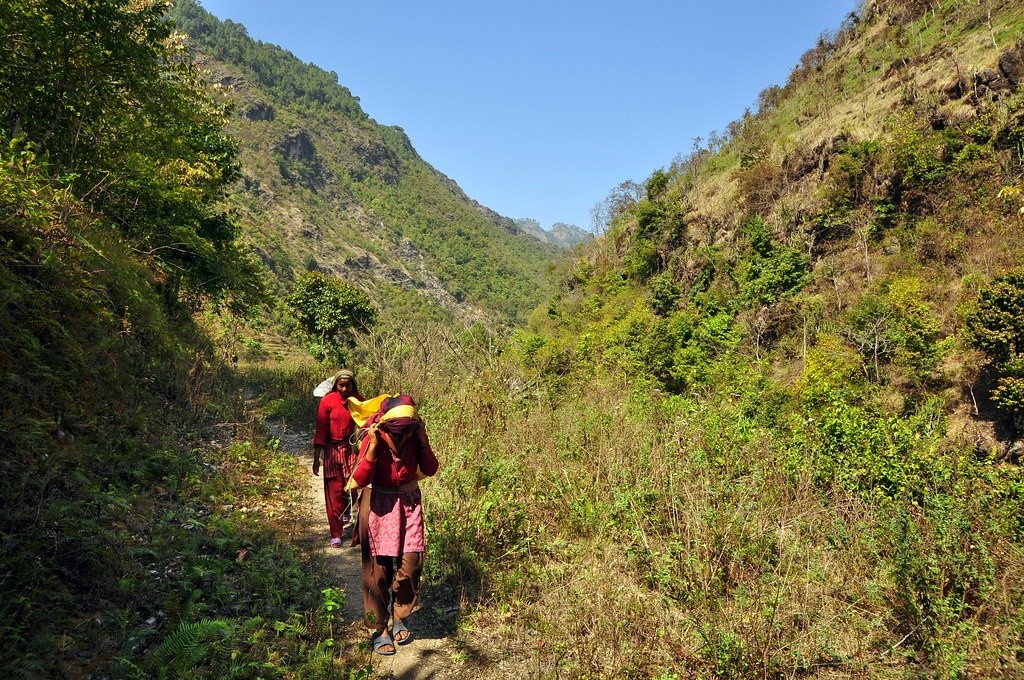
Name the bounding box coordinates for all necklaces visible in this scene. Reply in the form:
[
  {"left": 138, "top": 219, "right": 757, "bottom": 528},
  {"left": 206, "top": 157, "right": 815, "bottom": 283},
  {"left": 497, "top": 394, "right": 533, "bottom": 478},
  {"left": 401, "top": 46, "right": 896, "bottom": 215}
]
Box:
[{"left": 349, "top": 394, "right": 439, "bottom": 655}]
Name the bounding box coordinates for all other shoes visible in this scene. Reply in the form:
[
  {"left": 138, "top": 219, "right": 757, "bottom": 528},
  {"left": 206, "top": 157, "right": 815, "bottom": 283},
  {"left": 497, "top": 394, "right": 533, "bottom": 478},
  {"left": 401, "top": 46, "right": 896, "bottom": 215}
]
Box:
[{"left": 330, "top": 538, "right": 341, "bottom": 548}]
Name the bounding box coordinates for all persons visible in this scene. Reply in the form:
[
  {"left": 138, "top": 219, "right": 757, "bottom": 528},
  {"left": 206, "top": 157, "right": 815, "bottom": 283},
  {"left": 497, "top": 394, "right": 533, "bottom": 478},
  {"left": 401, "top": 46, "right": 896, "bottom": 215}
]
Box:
[{"left": 312, "top": 370, "right": 371, "bottom": 548}]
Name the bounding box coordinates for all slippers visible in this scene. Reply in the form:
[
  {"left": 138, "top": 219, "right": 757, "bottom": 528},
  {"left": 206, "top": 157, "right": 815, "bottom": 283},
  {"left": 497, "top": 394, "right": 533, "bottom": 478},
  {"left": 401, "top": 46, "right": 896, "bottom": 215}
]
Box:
[
  {"left": 372, "top": 632, "right": 395, "bottom": 654},
  {"left": 393, "top": 620, "right": 410, "bottom": 643}
]
